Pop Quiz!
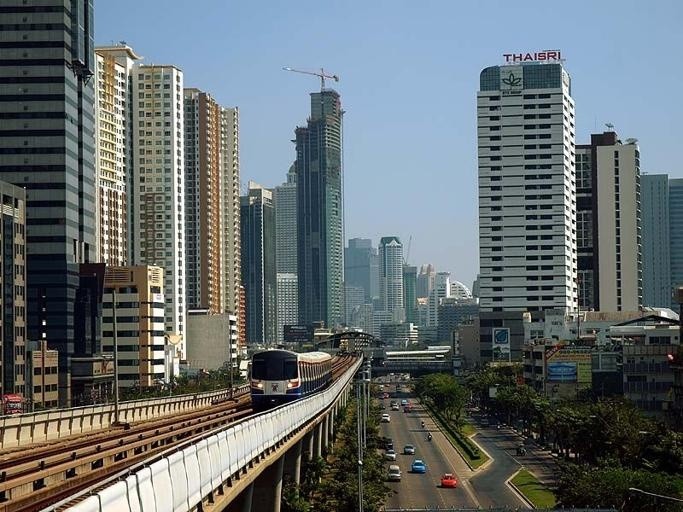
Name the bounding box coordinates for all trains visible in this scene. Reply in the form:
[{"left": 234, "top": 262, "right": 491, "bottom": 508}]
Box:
[{"left": 246, "top": 347, "right": 333, "bottom": 413}]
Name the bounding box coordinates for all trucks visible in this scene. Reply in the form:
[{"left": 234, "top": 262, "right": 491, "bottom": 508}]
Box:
[{"left": 2, "top": 394, "right": 22, "bottom": 416}]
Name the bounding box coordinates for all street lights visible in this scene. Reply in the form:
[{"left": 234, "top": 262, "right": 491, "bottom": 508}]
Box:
[
  {"left": 573, "top": 274, "right": 581, "bottom": 339},
  {"left": 350, "top": 351, "right": 373, "bottom": 512},
  {"left": 636, "top": 488, "right": 682, "bottom": 502},
  {"left": 160, "top": 333, "right": 182, "bottom": 395}
]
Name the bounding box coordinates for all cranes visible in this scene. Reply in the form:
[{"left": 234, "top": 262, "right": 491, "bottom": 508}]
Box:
[{"left": 282, "top": 64, "right": 338, "bottom": 89}]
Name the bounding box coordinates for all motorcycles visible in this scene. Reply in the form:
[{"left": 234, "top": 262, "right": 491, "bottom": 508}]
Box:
[{"left": 420, "top": 418, "right": 434, "bottom": 442}]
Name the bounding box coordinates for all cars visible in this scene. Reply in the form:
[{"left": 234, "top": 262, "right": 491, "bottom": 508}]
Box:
[
  {"left": 441, "top": 472, "right": 456, "bottom": 488},
  {"left": 403, "top": 444, "right": 415, "bottom": 455},
  {"left": 380, "top": 413, "right": 390, "bottom": 423},
  {"left": 385, "top": 449, "right": 397, "bottom": 462},
  {"left": 410, "top": 459, "right": 425, "bottom": 474},
  {"left": 376, "top": 373, "right": 412, "bottom": 413}
]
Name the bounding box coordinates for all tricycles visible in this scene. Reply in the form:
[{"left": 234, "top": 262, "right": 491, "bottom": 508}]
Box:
[{"left": 515, "top": 446, "right": 527, "bottom": 456}]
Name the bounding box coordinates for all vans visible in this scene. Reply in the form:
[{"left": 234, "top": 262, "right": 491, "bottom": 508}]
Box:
[
  {"left": 387, "top": 465, "right": 401, "bottom": 482},
  {"left": 383, "top": 438, "right": 393, "bottom": 451}
]
